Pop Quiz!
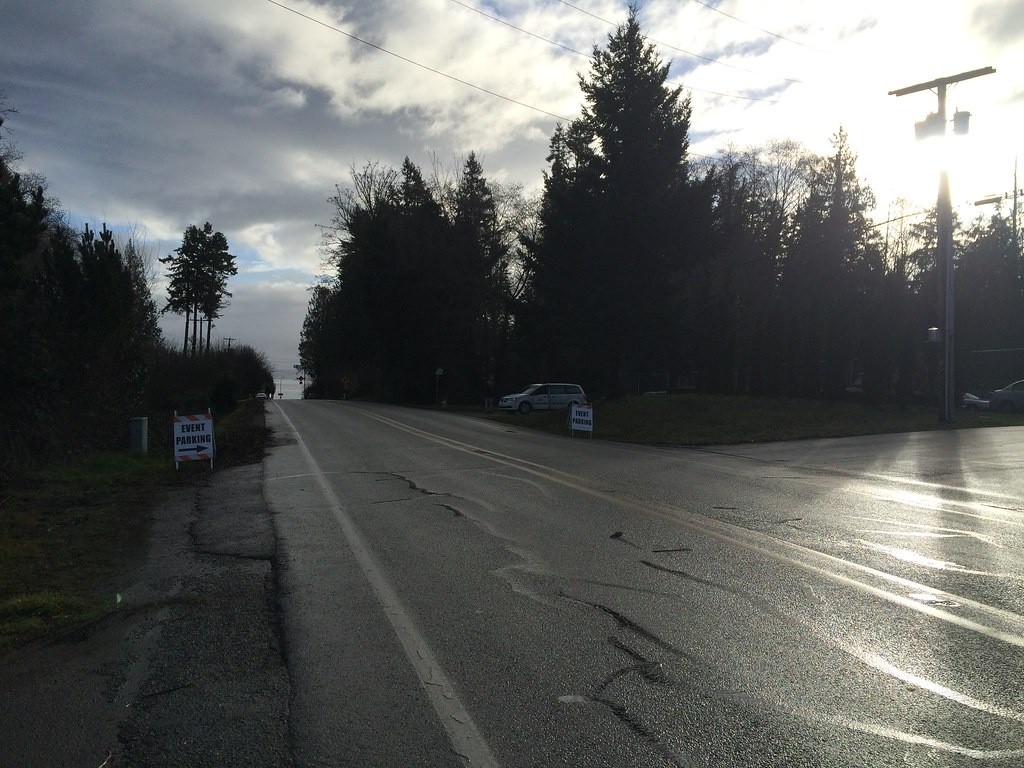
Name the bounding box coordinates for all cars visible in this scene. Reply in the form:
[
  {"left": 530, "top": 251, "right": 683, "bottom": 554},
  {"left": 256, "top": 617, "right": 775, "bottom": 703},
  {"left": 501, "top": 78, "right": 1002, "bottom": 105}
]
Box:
[
  {"left": 498, "top": 383, "right": 588, "bottom": 415},
  {"left": 962, "top": 392, "right": 991, "bottom": 411},
  {"left": 992, "top": 380, "right": 1023, "bottom": 415}
]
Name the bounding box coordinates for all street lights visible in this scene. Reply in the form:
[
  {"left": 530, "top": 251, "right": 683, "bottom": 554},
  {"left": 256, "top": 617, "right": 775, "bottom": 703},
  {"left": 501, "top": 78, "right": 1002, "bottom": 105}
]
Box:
[{"left": 279, "top": 376, "right": 286, "bottom": 400}]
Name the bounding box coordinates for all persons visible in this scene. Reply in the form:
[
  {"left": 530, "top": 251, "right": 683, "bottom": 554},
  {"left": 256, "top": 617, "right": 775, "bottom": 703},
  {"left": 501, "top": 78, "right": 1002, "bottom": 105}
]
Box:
[
  {"left": 309, "top": 393, "right": 312, "bottom": 400},
  {"left": 269, "top": 393, "right": 272, "bottom": 400}
]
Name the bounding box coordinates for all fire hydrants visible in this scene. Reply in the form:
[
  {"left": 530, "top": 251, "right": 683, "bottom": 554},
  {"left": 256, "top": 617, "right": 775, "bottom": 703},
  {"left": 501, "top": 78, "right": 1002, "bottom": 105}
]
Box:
[{"left": 441, "top": 399, "right": 448, "bottom": 407}]
